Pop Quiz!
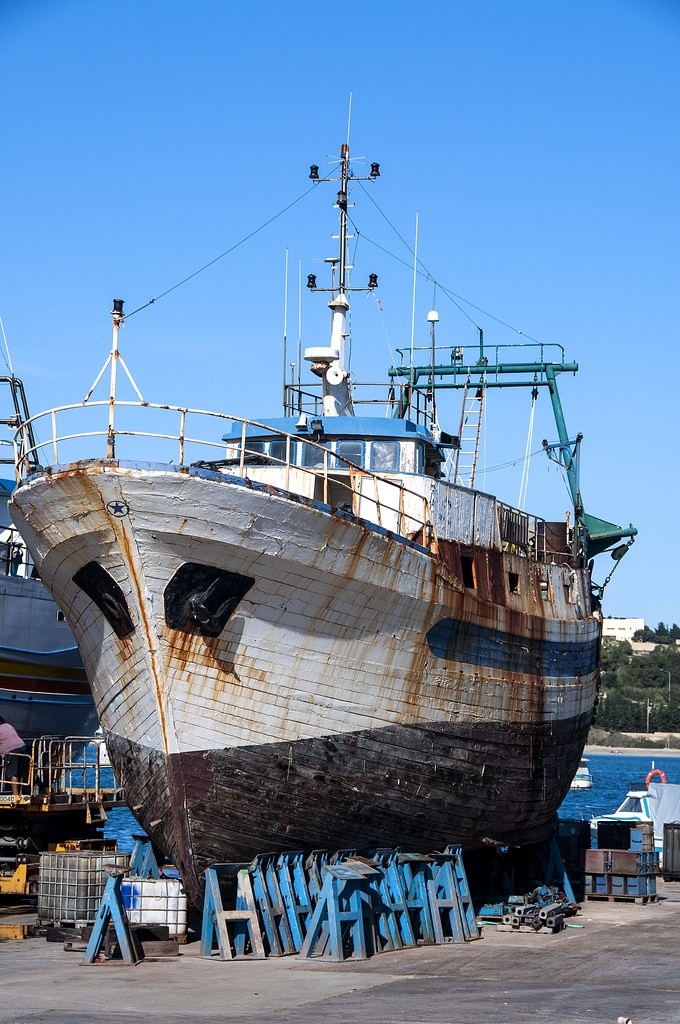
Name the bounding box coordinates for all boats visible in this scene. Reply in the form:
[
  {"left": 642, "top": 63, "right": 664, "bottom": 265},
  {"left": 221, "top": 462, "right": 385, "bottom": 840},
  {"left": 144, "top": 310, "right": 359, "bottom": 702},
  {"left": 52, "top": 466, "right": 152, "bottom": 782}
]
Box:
[
  {"left": 569, "top": 758, "right": 593, "bottom": 788},
  {"left": 0, "top": 318, "right": 99, "bottom": 789},
  {"left": 8, "top": 91, "right": 638, "bottom": 925},
  {"left": 590, "top": 760, "right": 680, "bottom": 869}
]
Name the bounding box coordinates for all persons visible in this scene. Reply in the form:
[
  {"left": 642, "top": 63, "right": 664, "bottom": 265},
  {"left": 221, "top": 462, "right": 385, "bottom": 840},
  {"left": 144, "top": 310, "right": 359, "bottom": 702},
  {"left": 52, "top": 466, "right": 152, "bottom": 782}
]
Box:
[{"left": 0, "top": 716, "right": 26, "bottom": 795}]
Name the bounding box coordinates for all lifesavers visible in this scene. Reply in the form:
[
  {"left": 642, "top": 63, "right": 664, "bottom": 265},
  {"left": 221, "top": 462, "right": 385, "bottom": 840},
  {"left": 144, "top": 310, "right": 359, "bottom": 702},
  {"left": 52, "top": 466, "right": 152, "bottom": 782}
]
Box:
[{"left": 645, "top": 769, "right": 667, "bottom": 789}]
